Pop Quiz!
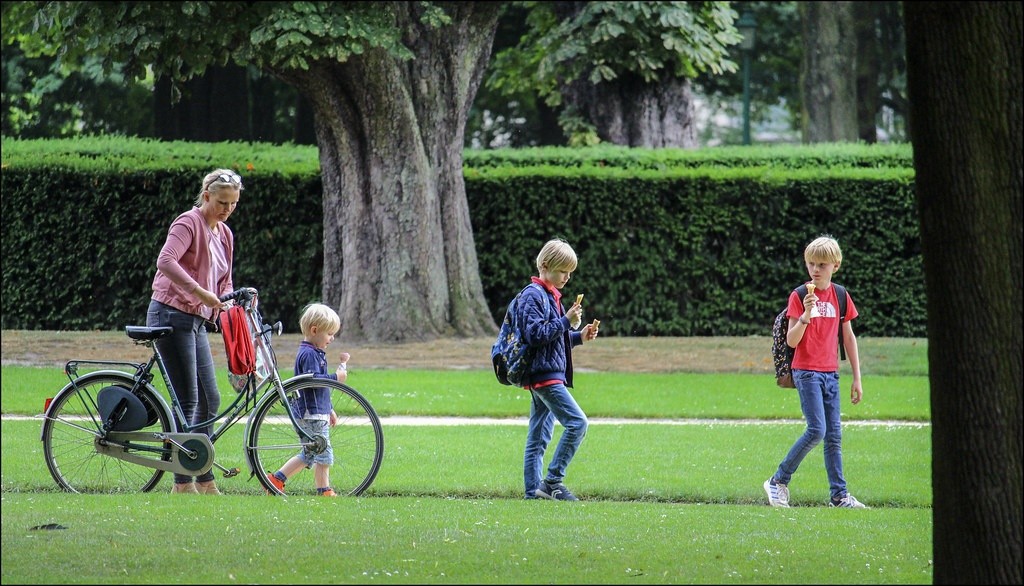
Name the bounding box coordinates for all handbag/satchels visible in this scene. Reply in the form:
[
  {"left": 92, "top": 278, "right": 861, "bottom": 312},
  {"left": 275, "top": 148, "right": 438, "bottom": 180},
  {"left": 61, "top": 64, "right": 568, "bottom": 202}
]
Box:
[
  {"left": 219, "top": 304, "right": 256, "bottom": 375},
  {"left": 228, "top": 299, "right": 270, "bottom": 394}
]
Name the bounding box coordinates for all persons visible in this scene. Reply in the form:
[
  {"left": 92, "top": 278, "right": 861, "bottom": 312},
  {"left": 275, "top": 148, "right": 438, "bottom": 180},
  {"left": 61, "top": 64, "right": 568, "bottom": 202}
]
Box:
[
  {"left": 147, "top": 166, "right": 245, "bottom": 496},
  {"left": 518, "top": 237, "right": 599, "bottom": 502},
  {"left": 765, "top": 234, "right": 867, "bottom": 511},
  {"left": 261, "top": 302, "right": 348, "bottom": 497}
]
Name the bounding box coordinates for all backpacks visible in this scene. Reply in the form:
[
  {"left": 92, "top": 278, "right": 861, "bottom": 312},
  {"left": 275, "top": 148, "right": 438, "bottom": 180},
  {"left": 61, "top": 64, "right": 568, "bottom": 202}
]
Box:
[
  {"left": 492, "top": 283, "right": 550, "bottom": 386},
  {"left": 772, "top": 284, "right": 847, "bottom": 389}
]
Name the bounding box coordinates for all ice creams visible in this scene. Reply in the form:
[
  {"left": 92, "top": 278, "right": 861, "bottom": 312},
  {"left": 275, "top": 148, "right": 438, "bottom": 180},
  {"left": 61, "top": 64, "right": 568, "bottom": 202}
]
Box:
[
  {"left": 576, "top": 294, "right": 584, "bottom": 305},
  {"left": 339, "top": 353, "right": 350, "bottom": 363},
  {"left": 806, "top": 284, "right": 816, "bottom": 294},
  {"left": 591, "top": 319, "right": 600, "bottom": 333}
]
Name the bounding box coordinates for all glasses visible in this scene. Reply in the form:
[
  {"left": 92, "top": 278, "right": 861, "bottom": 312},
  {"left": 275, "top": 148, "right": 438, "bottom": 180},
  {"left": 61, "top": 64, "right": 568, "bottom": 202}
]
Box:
[{"left": 206, "top": 174, "right": 241, "bottom": 190}]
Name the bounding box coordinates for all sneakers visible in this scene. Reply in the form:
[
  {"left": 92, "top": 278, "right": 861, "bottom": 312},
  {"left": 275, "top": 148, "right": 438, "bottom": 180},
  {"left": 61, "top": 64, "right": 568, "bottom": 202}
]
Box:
[
  {"left": 828, "top": 493, "right": 871, "bottom": 509},
  {"left": 764, "top": 476, "right": 790, "bottom": 508},
  {"left": 536, "top": 479, "right": 579, "bottom": 501},
  {"left": 317, "top": 488, "right": 337, "bottom": 497},
  {"left": 261, "top": 471, "right": 285, "bottom": 496}
]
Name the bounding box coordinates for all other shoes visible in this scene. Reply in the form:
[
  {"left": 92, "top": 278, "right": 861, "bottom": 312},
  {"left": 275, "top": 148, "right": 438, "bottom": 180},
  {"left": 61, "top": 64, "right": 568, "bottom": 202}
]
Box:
[{"left": 171, "top": 481, "right": 220, "bottom": 495}]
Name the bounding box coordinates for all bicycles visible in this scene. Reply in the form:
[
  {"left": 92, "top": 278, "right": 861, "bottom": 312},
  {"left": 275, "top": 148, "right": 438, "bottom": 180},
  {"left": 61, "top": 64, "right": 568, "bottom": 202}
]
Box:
[{"left": 40, "top": 288, "right": 385, "bottom": 497}]
